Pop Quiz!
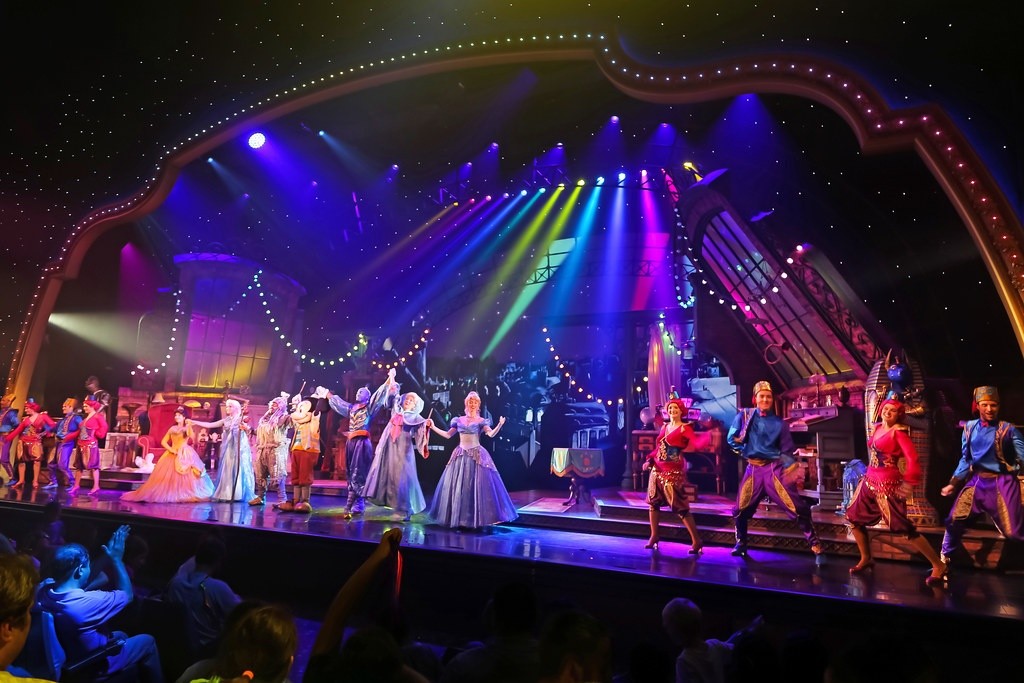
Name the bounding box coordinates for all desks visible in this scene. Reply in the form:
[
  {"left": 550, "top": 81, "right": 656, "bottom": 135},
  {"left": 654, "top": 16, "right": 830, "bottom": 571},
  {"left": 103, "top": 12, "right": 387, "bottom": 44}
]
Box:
[
  {"left": 549, "top": 447, "right": 604, "bottom": 506},
  {"left": 631, "top": 429, "right": 727, "bottom": 495}
]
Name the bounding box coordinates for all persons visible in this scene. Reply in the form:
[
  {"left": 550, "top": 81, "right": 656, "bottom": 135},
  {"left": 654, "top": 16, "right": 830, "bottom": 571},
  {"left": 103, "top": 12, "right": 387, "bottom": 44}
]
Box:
[
  {"left": 845, "top": 399, "right": 948, "bottom": 586},
  {"left": 36, "top": 399, "right": 83, "bottom": 492},
  {"left": 68, "top": 400, "right": 109, "bottom": 494},
  {"left": 425, "top": 391, "right": 519, "bottom": 535},
  {"left": 119, "top": 407, "right": 215, "bottom": 504},
  {"left": 240, "top": 398, "right": 294, "bottom": 506},
  {"left": 0, "top": 394, "right": 20, "bottom": 487},
  {"left": 184, "top": 399, "right": 253, "bottom": 503},
  {"left": 640, "top": 401, "right": 704, "bottom": 555},
  {"left": 174, "top": 602, "right": 296, "bottom": 683},
  {"left": 0, "top": 553, "right": 59, "bottom": 682},
  {"left": 84, "top": 371, "right": 113, "bottom": 433},
  {"left": 940, "top": 386, "right": 1024, "bottom": 564},
  {"left": 4, "top": 403, "right": 56, "bottom": 491},
  {"left": 538, "top": 597, "right": 942, "bottom": 683},
  {"left": 442, "top": 583, "right": 542, "bottom": 682},
  {"left": 725, "top": 381, "right": 828, "bottom": 570},
  {"left": 301, "top": 528, "right": 431, "bottom": 682},
  {"left": 0, "top": 500, "right": 243, "bottom": 683},
  {"left": 315, "top": 368, "right": 396, "bottom": 517},
  {"left": 361, "top": 368, "right": 430, "bottom": 521}
]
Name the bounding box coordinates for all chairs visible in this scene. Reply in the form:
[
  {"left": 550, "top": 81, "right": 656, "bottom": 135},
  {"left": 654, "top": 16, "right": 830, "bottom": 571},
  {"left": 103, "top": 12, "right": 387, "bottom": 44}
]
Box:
[{"left": 11, "top": 600, "right": 220, "bottom": 683}]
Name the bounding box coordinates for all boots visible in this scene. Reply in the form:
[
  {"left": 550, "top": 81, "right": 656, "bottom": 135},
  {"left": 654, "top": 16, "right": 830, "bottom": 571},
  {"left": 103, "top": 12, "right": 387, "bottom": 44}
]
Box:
[
  {"left": 644, "top": 506, "right": 660, "bottom": 549},
  {"left": 682, "top": 514, "right": 702, "bottom": 554}
]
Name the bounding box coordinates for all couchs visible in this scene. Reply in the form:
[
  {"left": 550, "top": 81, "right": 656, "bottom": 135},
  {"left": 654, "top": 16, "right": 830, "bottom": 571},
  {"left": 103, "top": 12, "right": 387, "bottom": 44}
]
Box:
[{"left": 137, "top": 402, "right": 194, "bottom": 464}]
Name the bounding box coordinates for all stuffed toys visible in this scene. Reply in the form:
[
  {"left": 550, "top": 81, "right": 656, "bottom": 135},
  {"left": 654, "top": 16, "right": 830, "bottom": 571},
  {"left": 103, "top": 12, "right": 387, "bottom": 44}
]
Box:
[{"left": 277, "top": 386, "right": 325, "bottom": 512}]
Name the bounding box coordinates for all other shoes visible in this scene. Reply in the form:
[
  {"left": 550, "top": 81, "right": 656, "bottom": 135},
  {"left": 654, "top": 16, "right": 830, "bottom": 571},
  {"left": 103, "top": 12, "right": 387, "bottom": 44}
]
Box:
[
  {"left": 816, "top": 554, "right": 826, "bottom": 567},
  {"left": 926, "top": 568, "right": 934, "bottom": 575},
  {"left": 272, "top": 502, "right": 283, "bottom": 508},
  {"left": 65, "top": 486, "right": 73, "bottom": 491},
  {"left": 731, "top": 543, "right": 747, "bottom": 556},
  {"left": 404, "top": 515, "right": 411, "bottom": 521},
  {"left": 41, "top": 484, "right": 58, "bottom": 490},
  {"left": 249, "top": 497, "right": 265, "bottom": 506},
  {"left": 5, "top": 480, "right": 17, "bottom": 486},
  {"left": 344, "top": 512, "right": 352, "bottom": 518}
]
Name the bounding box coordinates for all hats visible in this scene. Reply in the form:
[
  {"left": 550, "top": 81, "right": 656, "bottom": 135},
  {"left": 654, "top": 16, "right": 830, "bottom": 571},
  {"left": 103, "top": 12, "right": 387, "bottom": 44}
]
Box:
[
  {"left": 752, "top": 382, "right": 772, "bottom": 405},
  {"left": 85, "top": 395, "right": 94, "bottom": 400},
  {"left": 63, "top": 398, "right": 77, "bottom": 408},
  {"left": 6, "top": 394, "right": 16, "bottom": 402},
  {"left": 971, "top": 387, "right": 999, "bottom": 413}
]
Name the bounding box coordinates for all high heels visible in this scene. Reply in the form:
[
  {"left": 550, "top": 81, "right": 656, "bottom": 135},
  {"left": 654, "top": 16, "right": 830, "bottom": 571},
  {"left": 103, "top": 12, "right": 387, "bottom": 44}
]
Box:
[
  {"left": 848, "top": 561, "right": 875, "bottom": 575},
  {"left": 12, "top": 481, "right": 25, "bottom": 489},
  {"left": 32, "top": 480, "right": 38, "bottom": 488},
  {"left": 926, "top": 567, "right": 948, "bottom": 584},
  {"left": 69, "top": 486, "right": 80, "bottom": 493},
  {"left": 88, "top": 488, "right": 99, "bottom": 495}
]
